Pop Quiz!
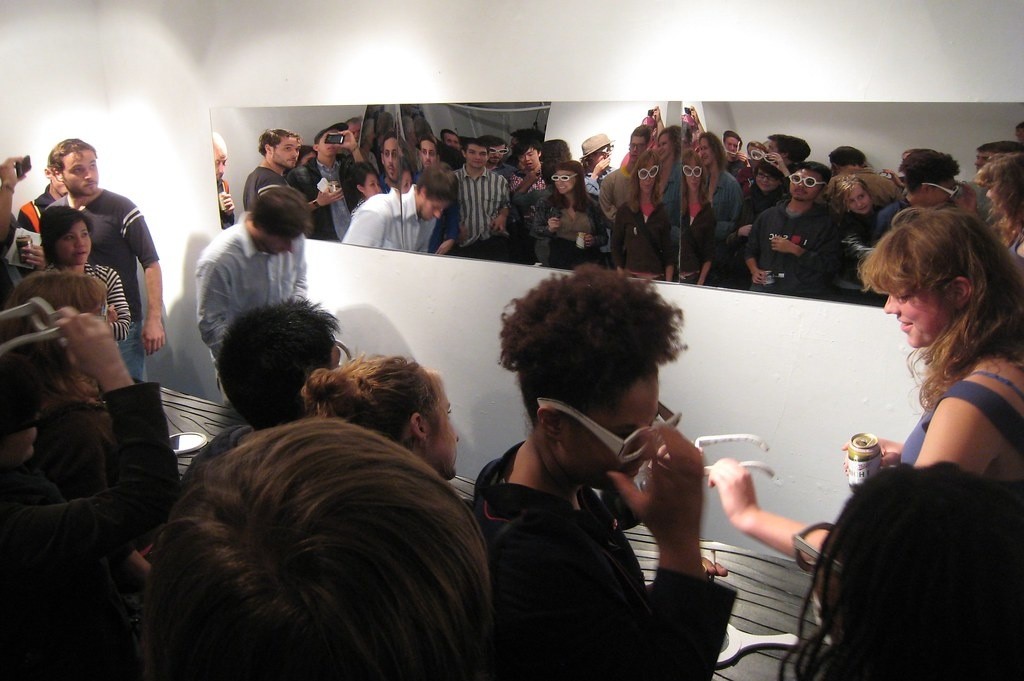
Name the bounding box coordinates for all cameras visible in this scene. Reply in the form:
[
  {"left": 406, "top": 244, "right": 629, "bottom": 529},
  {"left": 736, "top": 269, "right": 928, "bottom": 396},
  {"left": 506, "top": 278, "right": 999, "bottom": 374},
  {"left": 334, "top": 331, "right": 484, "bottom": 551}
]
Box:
[
  {"left": 648, "top": 110, "right": 655, "bottom": 116},
  {"left": 684, "top": 107, "right": 692, "bottom": 114},
  {"left": 329, "top": 181, "right": 339, "bottom": 193},
  {"left": 762, "top": 271, "right": 785, "bottom": 287}
]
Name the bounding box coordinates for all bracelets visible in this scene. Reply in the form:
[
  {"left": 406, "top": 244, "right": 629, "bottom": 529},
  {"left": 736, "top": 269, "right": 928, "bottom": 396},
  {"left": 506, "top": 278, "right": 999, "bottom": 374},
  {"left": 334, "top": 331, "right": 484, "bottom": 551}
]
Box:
[{"left": 312, "top": 200, "right": 322, "bottom": 208}]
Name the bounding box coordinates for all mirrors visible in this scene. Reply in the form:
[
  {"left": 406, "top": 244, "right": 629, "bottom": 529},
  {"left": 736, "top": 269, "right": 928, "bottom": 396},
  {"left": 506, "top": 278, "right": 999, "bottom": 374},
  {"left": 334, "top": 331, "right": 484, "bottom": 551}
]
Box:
[{"left": 208, "top": 102, "right": 1024, "bottom": 310}]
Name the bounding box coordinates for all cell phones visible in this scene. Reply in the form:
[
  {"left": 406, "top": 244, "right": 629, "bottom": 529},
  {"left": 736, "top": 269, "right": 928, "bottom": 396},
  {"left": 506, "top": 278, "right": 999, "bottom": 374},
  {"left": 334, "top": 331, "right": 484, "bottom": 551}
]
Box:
[
  {"left": 15, "top": 155, "right": 31, "bottom": 179},
  {"left": 324, "top": 134, "right": 344, "bottom": 145}
]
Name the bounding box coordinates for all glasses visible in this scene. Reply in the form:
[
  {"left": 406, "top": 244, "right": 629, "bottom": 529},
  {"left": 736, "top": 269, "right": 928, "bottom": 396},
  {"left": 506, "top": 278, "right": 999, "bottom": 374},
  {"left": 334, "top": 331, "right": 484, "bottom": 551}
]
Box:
[
  {"left": 333, "top": 340, "right": 351, "bottom": 362},
  {"left": 694, "top": 432, "right": 774, "bottom": 476},
  {"left": 793, "top": 524, "right": 842, "bottom": 582},
  {"left": 683, "top": 164, "right": 704, "bottom": 178},
  {"left": 750, "top": 150, "right": 777, "bottom": 163},
  {"left": 552, "top": 173, "right": 578, "bottom": 181},
  {"left": 534, "top": 391, "right": 682, "bottom": 462},
  {"left": 788, "top": 173, "right": 828, "bottom": 188},
  {"left": 0, "top": 299, "right": 79, "bottom": 358},
  {"left": 720, "top": 135, "right": 741, "bottom": 157},
  {"left": 637, "top": 168, "right": 660, "bottom": 180},
  {"left": 922, "top": 182, "right": 959, "bottom": 198},
  {"left": 488, "top": 147, "right": 509, "bottom": 155},
  {"left": 595, "top": 145, "right": 615, "bottom": 156}
]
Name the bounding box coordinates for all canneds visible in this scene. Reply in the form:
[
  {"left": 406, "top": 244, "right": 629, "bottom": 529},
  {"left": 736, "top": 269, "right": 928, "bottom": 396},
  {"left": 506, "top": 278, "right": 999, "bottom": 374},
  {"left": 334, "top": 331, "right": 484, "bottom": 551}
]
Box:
[
  {"left": 16, "top": 236, "right": 35, "bottom": 263},
  {"left": 847, "top": 433, "right": 882, "bottom": 493},
  {"left": 329, "top": 180, "right": 339, "bottom": 193},
  {"left": 761, "top": 271, "right": 775, "bottom": 293},
  {"left": 576, "top": 232, "right": 587, "bottom": 249},
  {"left": 219, "top": 191, "right": 229, "bottom": 210}
]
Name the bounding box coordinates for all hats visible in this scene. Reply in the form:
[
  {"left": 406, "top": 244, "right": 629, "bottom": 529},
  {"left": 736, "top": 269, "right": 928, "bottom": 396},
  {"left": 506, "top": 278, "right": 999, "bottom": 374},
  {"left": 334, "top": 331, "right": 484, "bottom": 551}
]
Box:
[
  {"left": 579, "top": 135, "right": 616, "bottom": 158},
  {"left": 314, "top": 121, "right": 348, "bottom": 143}
]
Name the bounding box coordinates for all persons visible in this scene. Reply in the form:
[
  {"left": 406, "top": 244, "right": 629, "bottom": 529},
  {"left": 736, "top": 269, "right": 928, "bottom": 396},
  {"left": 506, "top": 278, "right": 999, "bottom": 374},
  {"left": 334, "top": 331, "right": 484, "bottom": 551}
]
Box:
[
  {"left": 195, "top": 183, "right": 313, "bottom": 406},
  {"left": 0, "top": 139, "right": 1023, "bottom": 681},
  {"left": 209, "top": 106, "right": 1024, "bottom": 308},
  {"left": 473, "top": 262, "right": 738, "bottom": 681}
]
